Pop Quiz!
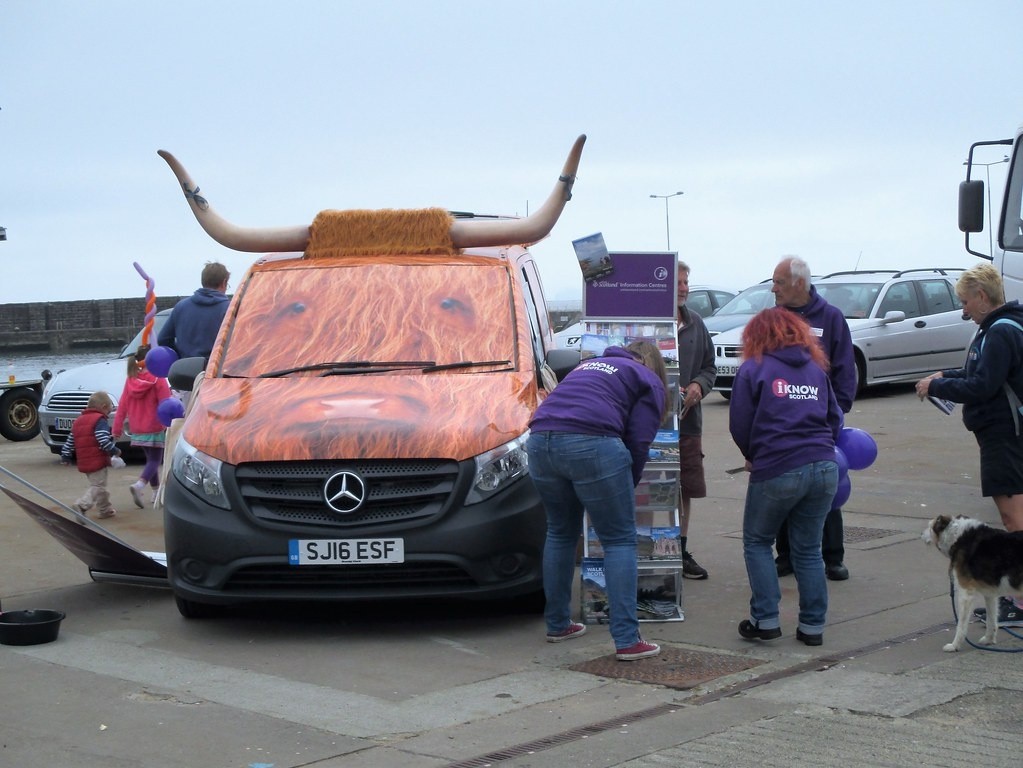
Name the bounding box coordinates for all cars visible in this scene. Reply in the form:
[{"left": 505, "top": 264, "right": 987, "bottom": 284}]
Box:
[
  {"left": 37, "top": 308, "right": 173, "bottom": 459},
  {"left": 554, "top": 285, "right": 741, "bottom": 377}
]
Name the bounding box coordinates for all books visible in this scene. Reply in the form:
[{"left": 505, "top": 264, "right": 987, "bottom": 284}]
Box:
[
  {"left": 583, "top": 320, "right": 680, "bottom": 559},
  {"left": 572, "top": 232, "right": 616, "bottom": 283}
]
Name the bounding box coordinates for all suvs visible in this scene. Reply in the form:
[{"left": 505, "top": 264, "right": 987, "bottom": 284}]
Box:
[{"left": 699, "top": 268, "right": 981, "bottom": 401}]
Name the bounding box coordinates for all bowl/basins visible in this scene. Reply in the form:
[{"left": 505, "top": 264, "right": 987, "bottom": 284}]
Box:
[{"left": 0, "top": 608, "right": 66, "bottom": 646}]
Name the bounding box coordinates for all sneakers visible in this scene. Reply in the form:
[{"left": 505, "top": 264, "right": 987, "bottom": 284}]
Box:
[
  {"left": 677, "top": 550, "right": 708, "bottom": 580},
  {"left": 738, "top": 619, "right": 782, "bottom": 640},
  {"left": 796, "top": 627, "right": 823, "bottom": 646},
  {"left": 615, "top": 640, "right": 660, "bottom": 660},
  {"left": 973, "top": 595, "right": 1023, "bottom": 629},
  {"left": 546, "top": 619, "right": 587, "bottom": 642}
]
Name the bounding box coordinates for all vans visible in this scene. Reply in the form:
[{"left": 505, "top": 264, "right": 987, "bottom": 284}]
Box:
[{"left": 164, "top": 212, "right": 552, "bottom": 619}]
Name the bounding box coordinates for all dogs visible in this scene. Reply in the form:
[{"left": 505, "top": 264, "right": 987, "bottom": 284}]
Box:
[{"left": 919, "top": 512, "right": 1023, "bottom": 653}]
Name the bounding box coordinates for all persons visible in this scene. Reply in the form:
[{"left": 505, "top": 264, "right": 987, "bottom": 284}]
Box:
[
  {"left": 771, "top": 257, "right": 856, "bottom": 581},
  {"left": 648, "top": 261, "right": 717, "bottom": 580},
  {"left": 527, "top": 342, "right": 668, "bottom": 660},
  {"left": 916, "top": 266, "right": 1023, "bottom": 626},
  {"left": 60, "top": 391, "right": 126, "bottom": 525},
  {"left": 158, "top": 262, "right": 235, "bottom": 371},
  {"left": 112, "top": 344, "right": 170, "bottom": 508},
  {"left": 729, "top": 308, "right": 843, "bottom": 646}
]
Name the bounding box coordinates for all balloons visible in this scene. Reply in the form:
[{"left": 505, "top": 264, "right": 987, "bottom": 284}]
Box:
[
  {"left": 156, "top": 397, "right": 185, "bottom": 427},
  {"left": 133, "top": 260, "right": 157, "bottom": 350},
  {"left": 832, "top": 426, "right": 877, "bottom": 508},
  {"left": 145, "top": 346, "right": 180, "bottom": 380}
]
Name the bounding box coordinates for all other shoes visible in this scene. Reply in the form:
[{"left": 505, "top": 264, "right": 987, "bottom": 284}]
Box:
[
  {"left": 825, "top": 559, "right": 849, "bottom": 581},
  {"left": 151, "top": 495, "right": 157, "bottom": 502},
  {"left": 130, "top": 483, "right": 144, "bottom": 509},
  {"left": 72, "top": 504, "right": 87, "bottom": 525},
  {"left": 97, "top": 508, "right": 117, "bottom": 519},
  {"left": 774, "top": 554, "right": 793, "bottom": 577}
]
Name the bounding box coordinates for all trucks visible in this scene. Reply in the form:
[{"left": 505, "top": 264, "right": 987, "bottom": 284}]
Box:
[{"left": 958, "top": 123, "right": 1023, "bottom": 304}]
